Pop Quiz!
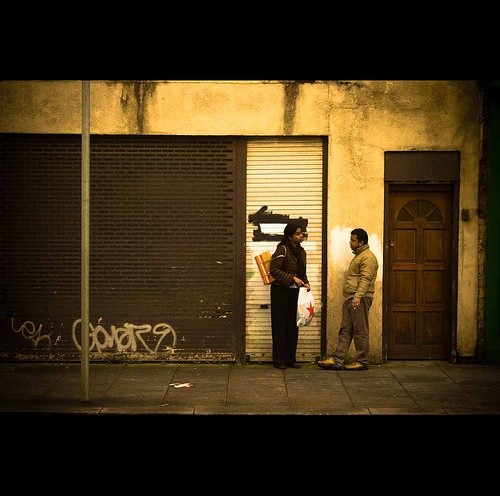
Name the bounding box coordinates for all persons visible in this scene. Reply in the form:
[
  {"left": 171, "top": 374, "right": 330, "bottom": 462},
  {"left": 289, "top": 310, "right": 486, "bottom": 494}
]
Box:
[
  {"left": 316, "top": 228, "right": 379, "bottom": 371},
  {"left": 270, "top": 222, "right": 311, "bottom": 369}
]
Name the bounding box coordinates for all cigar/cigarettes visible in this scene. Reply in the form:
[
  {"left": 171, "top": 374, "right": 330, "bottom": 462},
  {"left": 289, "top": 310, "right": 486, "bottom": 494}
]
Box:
[{"left": 354, "top": 307, "right": 357, "bottom": 310}]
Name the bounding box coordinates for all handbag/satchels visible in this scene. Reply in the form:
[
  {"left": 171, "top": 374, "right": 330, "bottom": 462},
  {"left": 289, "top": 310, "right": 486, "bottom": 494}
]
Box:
[
  {"left": 254, "top": 245, "right": 287, "bottom": 285},
  {"left": 296, "top": 287, "right": 315, "bottom": 327}
]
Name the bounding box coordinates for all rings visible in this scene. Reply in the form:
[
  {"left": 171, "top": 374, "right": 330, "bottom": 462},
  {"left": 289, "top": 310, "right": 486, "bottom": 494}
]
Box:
[{"left": 357, "top": 304, "right": 358, "bottom": 305}]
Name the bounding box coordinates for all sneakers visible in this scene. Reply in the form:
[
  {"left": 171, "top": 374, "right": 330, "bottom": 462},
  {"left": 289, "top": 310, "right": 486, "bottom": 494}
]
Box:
[
  {"left": 344, "top": 360, "right": 368, "bottom": 370},
  {"left": 318, "top": 356, "right": 343, "bottom": 369}
]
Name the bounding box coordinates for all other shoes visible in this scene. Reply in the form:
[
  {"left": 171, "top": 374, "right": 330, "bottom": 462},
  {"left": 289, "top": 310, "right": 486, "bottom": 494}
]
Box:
[
  {"left": 286, "top": 364, "right": 302, "bottom": 368},
  {"left": 273, "top": 364, "right": 287, "bottom": 369}
]
